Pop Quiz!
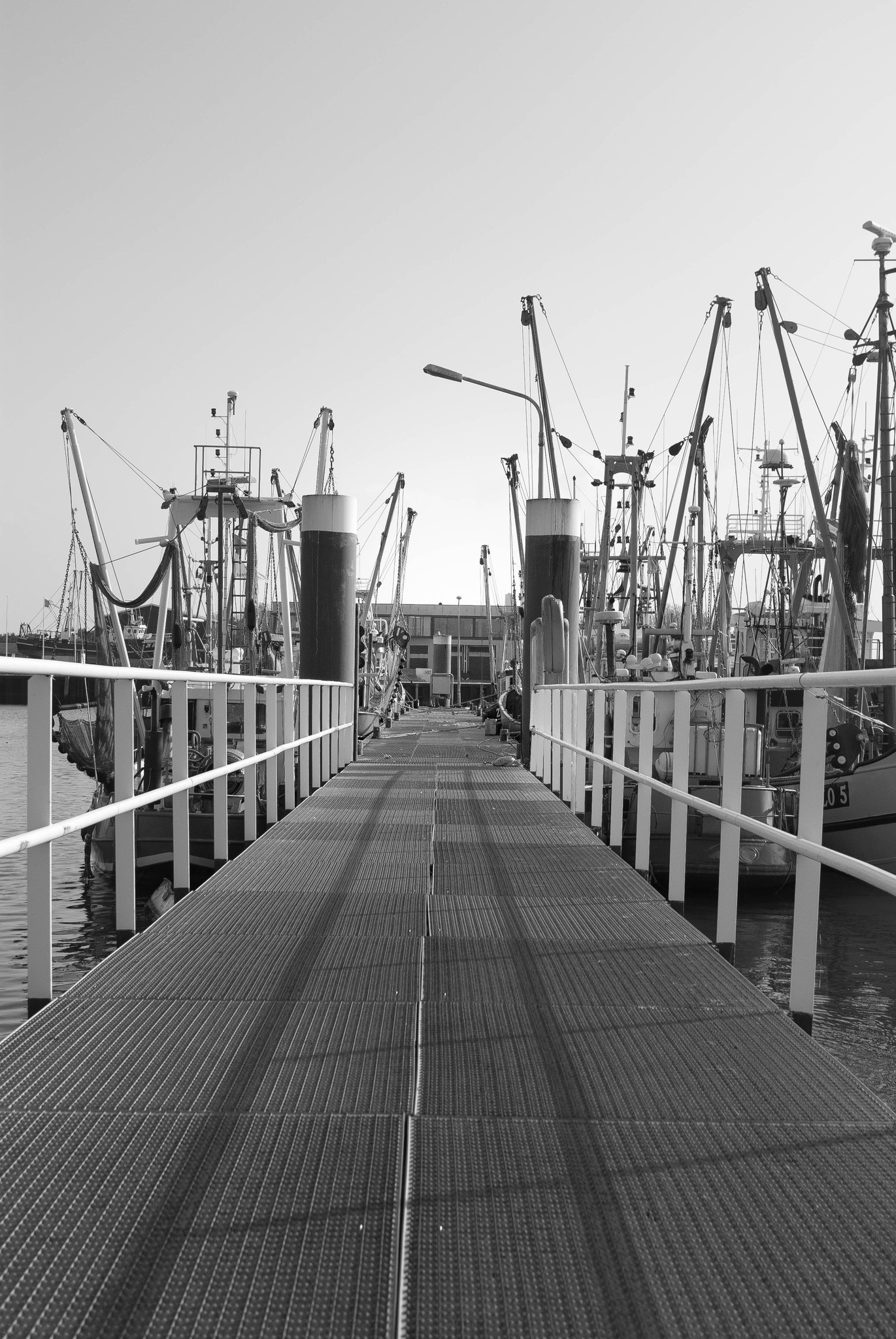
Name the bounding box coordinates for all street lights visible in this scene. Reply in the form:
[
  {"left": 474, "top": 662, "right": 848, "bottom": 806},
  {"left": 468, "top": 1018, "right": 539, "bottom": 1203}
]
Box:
[{"left": 424, "top": 364, "right": 545, "bottom": 498}]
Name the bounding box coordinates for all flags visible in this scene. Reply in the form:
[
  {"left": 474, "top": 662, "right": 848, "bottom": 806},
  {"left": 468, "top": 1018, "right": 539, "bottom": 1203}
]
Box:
[{"left": 45, "top": 599, "right": 49, "bottom": 607}]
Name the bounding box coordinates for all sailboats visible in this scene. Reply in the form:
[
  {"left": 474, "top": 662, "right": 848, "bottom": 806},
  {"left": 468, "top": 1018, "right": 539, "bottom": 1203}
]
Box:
[
  {"left": 17, "top": 390, "right": 419, "bottom": 880},
  {"left": 479, "top": 221, "right": 895, "bottom": 892}
]
